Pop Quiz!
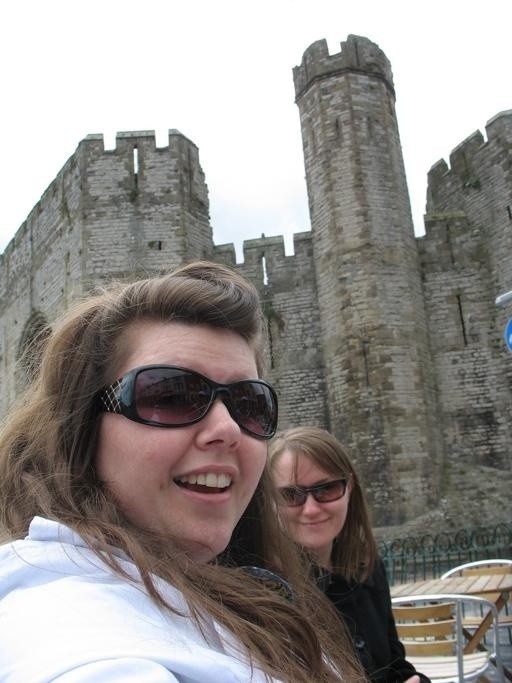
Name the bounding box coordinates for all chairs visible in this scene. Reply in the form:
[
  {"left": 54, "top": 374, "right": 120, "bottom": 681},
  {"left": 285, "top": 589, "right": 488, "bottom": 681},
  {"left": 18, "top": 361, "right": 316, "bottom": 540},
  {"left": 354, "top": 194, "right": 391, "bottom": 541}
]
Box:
[{"left": 387, "top": 557, "right": 512, "bottom": 681}]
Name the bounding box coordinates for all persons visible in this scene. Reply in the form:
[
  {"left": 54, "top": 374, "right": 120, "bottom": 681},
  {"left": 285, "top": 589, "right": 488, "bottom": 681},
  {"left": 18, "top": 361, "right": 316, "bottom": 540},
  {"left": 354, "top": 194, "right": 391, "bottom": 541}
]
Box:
[
  {"left": 257, "top": 423, "right": 432, "bottom": 682},
  {"left": 1, "top": 255, "right": 377, "bottom": 682}
]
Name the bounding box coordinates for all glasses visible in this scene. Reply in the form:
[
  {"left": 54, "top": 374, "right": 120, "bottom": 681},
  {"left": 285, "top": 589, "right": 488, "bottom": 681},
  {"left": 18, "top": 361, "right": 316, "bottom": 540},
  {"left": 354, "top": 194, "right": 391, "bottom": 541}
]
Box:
[
  {"left": 95, "top": 364, "right": 278, "bottom": 441},
  {"left": 276, "top": 473, "right": 353, "bottom": 508}
]
